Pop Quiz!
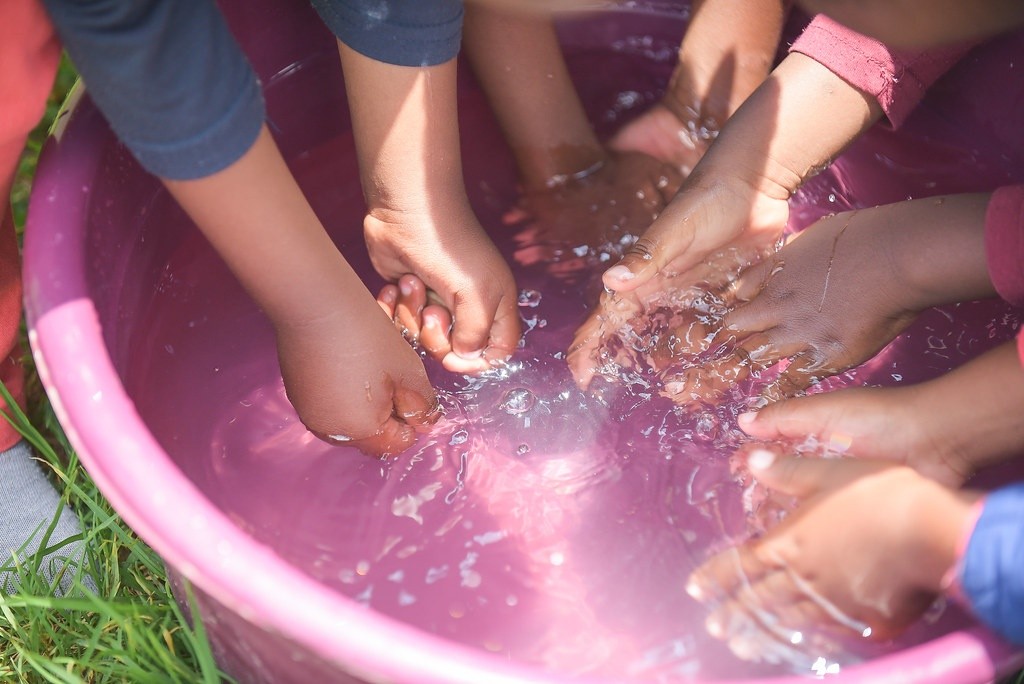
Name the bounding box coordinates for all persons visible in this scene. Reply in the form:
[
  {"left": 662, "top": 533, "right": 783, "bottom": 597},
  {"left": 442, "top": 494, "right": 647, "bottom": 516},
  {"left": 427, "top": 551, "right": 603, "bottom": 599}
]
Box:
[
  {"left": 460, "top": 0, "right": 1024, "bottom": 660},
  {"left": 0, "top": 0, "right": 524, "bottom": 604}
]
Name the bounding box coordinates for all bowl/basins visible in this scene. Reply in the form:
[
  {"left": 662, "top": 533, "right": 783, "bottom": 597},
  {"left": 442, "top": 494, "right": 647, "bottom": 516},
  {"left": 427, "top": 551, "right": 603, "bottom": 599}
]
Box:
[{"left": 20, "top": 1, "right": 1024, "bottom": 684}]
